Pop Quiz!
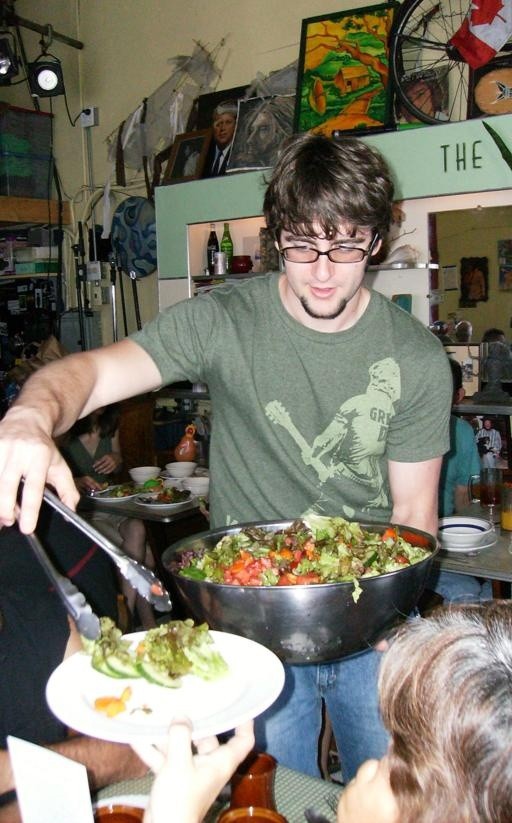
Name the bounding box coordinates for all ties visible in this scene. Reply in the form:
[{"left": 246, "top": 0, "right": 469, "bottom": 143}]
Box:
[{"left": 212, "top": 152, "right": 222, "bottom": 177}]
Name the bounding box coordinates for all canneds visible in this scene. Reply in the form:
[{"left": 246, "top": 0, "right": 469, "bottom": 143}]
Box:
[{"left": 214, "top": 252, "right": 228, "bottom": 274}]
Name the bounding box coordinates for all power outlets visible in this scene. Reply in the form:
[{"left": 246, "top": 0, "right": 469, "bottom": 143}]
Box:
[{"left": 80, "top": 105, "right": 99, "bottom": 127}]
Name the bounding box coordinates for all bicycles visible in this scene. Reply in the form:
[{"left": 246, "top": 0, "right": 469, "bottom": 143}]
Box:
[{"left": 385, "top": 0, "right": 512, "bottom": 126}]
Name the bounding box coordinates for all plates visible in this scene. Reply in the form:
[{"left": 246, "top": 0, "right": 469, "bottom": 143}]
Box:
[
  {"left": 89, "top": 460, "right": 212, "bottom": 511},
  {"left": 433, "top": 533, "right": 500, "bottom": 551},
  {"left": 45, "top": 620, "right": 290, "bottom": 743}
]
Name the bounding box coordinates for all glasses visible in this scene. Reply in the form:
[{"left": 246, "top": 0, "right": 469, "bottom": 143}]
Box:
[{"left": 278, "top": 233, "right": 378, "bottom": 263}]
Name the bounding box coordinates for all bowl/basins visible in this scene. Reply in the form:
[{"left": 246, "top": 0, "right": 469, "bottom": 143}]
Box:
[
  {"left": 230, "top": 255, "right": 255, "bottom": 271},
  {"left": 437, "top": 516, "right": 493, "bottom": 546},
  {"left": 164, "top": 515, "right": 446, "bottom": 666}
]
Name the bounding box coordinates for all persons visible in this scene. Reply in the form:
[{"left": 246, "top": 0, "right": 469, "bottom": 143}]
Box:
[
  {"left": 338, "top": 597, "right": 512, "bottom": 823},
  {"left": 243, "top": 97, "right": 296, "bottom": 166},
  {"left": 0, "top": 131, "right": 453, "bottom": 787},
  {"left": 201, "top": 98, "right": 237, "bottom": 178},
  {"left": 129, "top": 714, "right": 255, "bottom": 823},
  {"left": 0, "top": 477, "right": 151, "bottom": 802},
  {"left": 53, "top": 405, "right": 158, "bottom": 631},
  {"left": 462, "top": 259, "right": 485, "bottom": 300},
  {"left": 437, "top": 357, "right": 483, "bottom": 517},
  {"left": 475, "top": 419, "right": 502, "bottom": 468},
  {"left": 182, "top": 140, "right": 201, "bottom": 176}
]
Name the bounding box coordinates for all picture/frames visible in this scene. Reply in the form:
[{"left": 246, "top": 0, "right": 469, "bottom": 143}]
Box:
[
  {"left": 402, "top": 58, "right": 453, "bottom": 131},
  {"left": 183, "top": 83, "right": 266, "bottom": 177},
  {"left": 160, "top": 122, "right": 216, "bottom": 186},
  {"left": 288, "top": 0, "right": 399, "bottom": 140}
]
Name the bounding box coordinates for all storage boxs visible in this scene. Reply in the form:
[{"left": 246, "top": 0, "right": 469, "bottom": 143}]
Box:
[
  {"left": 0, "top": 102, "right": 55, "bottom": 157},
  {"left": 0, "top": 149, "right": 58, "bottom": 200}
]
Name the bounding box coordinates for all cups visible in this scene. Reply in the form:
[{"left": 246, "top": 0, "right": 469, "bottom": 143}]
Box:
[
  {"left": 217, "top": 807, "right": 287, "bottom": 823},
  {"left": 227, "top": 753, "right": 281, "bottom": 810},
  {"left": 467, "top": 465, "right": 500, "bottom": 509},
  {"left": 96, "top": 802, "right": 144, "bottom": 821}
]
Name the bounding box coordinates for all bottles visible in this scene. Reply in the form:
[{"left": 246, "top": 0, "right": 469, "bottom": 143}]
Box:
[{"left": 203, "top": 222, "right": 235, "bottom": 273}]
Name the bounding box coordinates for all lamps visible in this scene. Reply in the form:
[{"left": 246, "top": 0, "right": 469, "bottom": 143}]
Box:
[{"left": 0, "top": 9, "right": 92, "bottom": 107}]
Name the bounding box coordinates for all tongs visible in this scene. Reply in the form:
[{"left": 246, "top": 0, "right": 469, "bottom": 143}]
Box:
[{"left": 8, "top": 474, "right": 176, "bottom": 645}]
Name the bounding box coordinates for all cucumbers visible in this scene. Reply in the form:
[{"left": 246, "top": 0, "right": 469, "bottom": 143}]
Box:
[{"left": 91, "top": 641, "right": 183, "bottom": 690}]
